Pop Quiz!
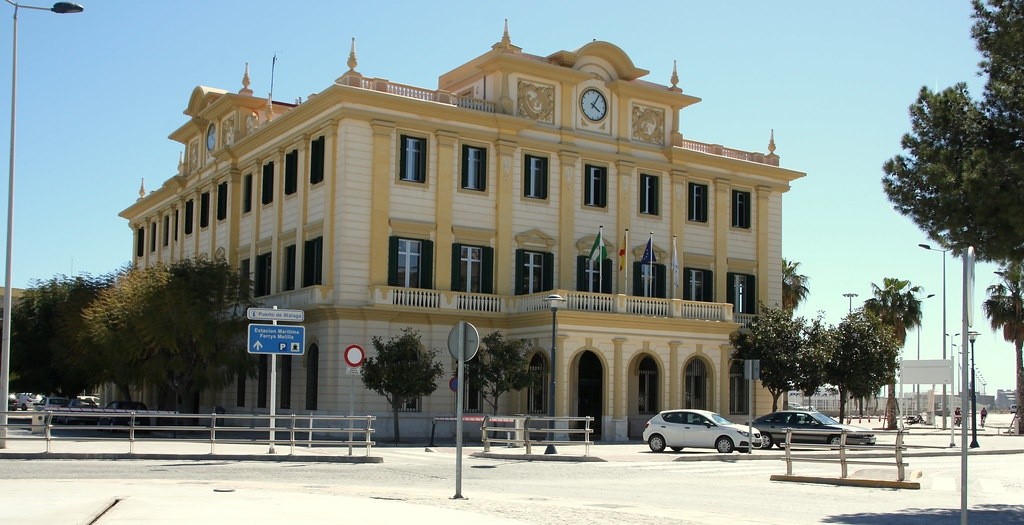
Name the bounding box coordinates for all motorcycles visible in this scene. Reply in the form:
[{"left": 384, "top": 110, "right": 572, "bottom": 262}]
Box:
[{"left": 953, "top": 411, "right": 964, "bottom": 427}]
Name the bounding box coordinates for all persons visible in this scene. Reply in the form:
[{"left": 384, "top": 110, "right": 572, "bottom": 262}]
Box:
[
  {"left": 980, "top": 406, "right": 987, "bottom": 426},
  {"left": 954, "top": 407, "right": 962, "bottom": 424}
]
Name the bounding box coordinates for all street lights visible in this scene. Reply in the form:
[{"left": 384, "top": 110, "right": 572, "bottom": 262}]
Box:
[
  {"left": 1, "top": 0, "right": 85, "bottom": 448},
  {"left": 541, "top": 295, "right": 567, "bottom": 453},
  {"left": 917, "top": 243, "right": 953, "bottom": 430}
]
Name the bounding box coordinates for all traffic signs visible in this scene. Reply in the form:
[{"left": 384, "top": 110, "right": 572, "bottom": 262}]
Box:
[{"left": 246, "top": 323, "right": 307, "bottom": 356}]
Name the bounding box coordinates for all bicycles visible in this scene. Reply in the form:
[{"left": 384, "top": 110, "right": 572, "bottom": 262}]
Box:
[{"left": 979, "top": 412, "right": 987, "bottom": 427}]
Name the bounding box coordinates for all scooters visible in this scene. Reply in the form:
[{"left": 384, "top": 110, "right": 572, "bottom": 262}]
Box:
[{"left": 906, "top": 414, "right": 926, "bottom": 425}]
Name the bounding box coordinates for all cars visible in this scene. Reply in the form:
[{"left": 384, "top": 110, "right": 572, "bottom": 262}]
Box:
[
  {"left": 76, "top": 395, "right": 101, "bottom": 407},
  {"left": 743, "top": 409, "right": 877, "bottom": 453},
  {"left": 98, "top": 400, "right": 149, "bottom": 434},
  {"left": 641, "top": 408, "right": 764, "bottom": 454},
  {"left": 8, "top": 392, "right": 18, "bottom": 410},
  {"left": 33, "top": 396, "right": 70, "bottom": 422},
  {"left": 16, "top": 392, "right": 45, "bottom": 412},
  {"left": 58, "top": 398, "right": 100, "bottom": 428}
]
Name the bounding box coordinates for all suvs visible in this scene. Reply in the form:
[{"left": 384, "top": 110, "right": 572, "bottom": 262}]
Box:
[
  {"left": 787, "top": 401, "right": 803, "bottom": 411},
  {"left": 935, "top": 406, "right": 950, "bottom": 416}
]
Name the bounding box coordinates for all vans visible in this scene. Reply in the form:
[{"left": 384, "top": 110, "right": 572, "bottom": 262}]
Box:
[{"left": 1010, "top": 403, "right": 1018, "bottom": 413}]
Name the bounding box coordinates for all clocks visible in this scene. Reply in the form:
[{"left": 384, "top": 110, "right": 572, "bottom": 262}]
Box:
[
  {"left": 580, "top": 86, "right": 609, "bottom": 124},
  {"left": 206, "top": 123, "right": 216, "bottom": 150}
]
